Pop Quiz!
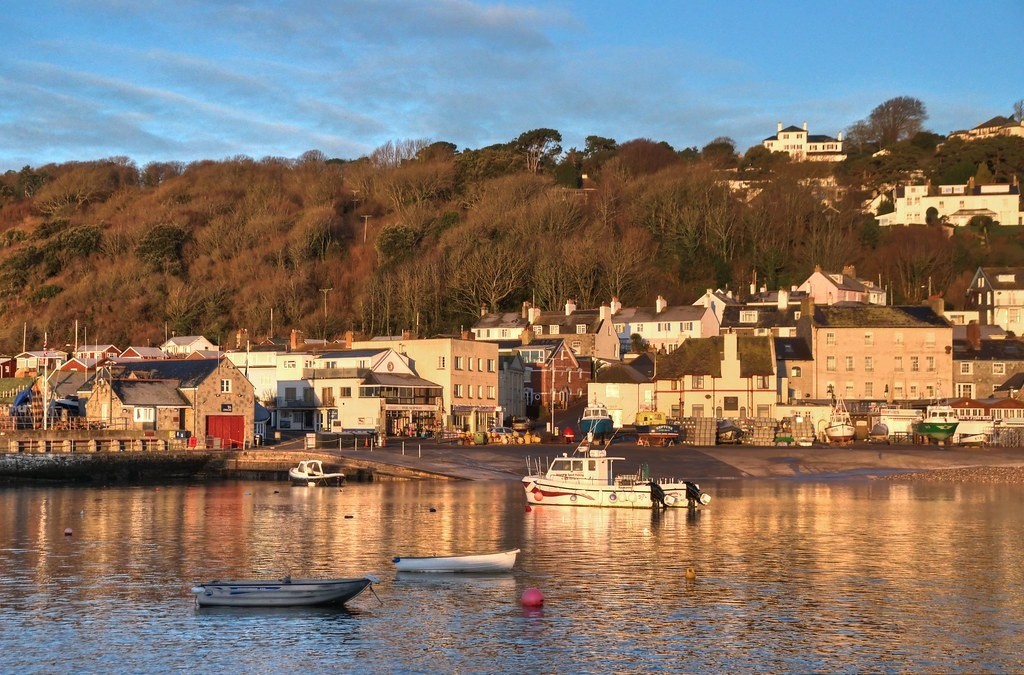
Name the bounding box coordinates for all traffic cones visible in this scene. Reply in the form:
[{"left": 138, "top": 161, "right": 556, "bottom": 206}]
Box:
[{"left": 365, "top": 437, "right": 369, "bottom": 447}]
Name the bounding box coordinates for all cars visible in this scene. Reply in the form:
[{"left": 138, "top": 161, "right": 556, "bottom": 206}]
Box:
[
  {"left": 486, "top": 426, "right": 518, "bottom": 439},
  {"left": 514, "top": 417, "right": 534, "bottom": 431}
]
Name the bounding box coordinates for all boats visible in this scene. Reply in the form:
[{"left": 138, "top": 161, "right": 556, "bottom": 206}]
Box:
[
  {"left": 289, "top": 459, "right": 345, "bottom": 487},
  {"left": 191, "top": 575, "right": 381, "bottom": 608},
  {"left": 717, "top": 419, "right": 743, "bottom": 443},
  {"left": 823, "top": 392, "right": 856, "bottom": 442},
  {"left": 392, "top": 547, "right": 521, "bottom": 573},
  {"left": 961, "top": 433, "right": 989, "bottom": 448},
  {"left": 910, "top": 421, "right": 960, "bottom": 444},
  {"left": 521, "top": 433, "right": 711, "bottom": 508},
  {"left": 580, "top": 406, "right": 615, "bottom": 435}
]
